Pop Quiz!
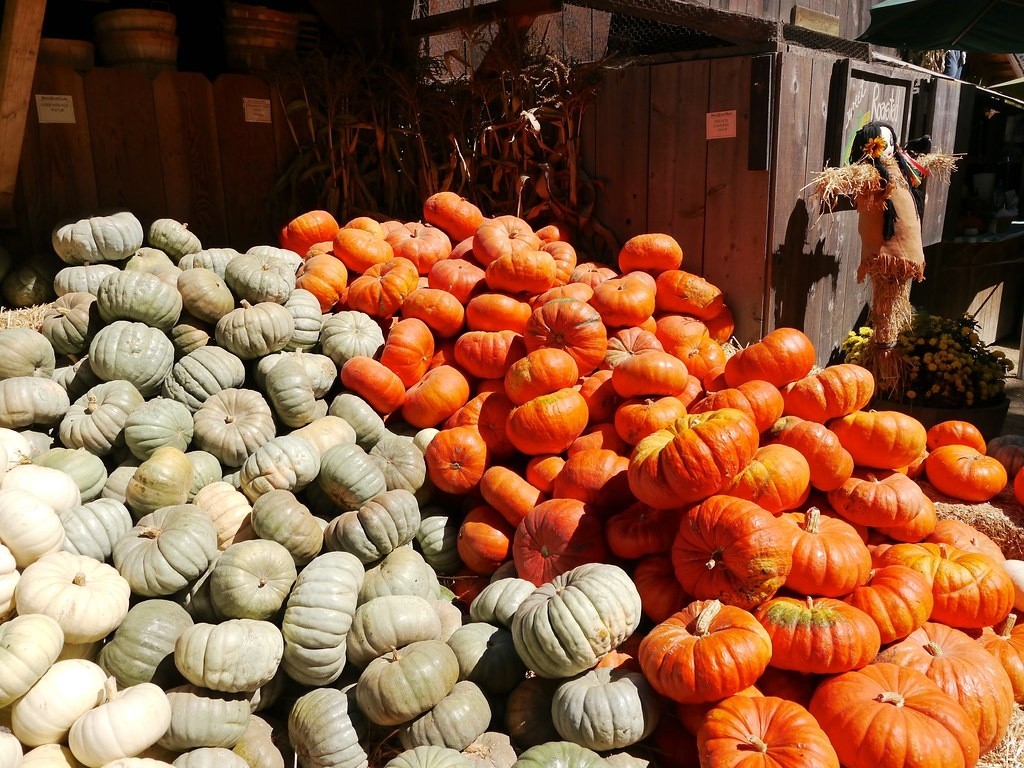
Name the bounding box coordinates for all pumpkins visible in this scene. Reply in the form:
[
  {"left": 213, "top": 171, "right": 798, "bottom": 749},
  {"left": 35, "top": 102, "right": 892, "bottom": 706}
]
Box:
[
  {"left": 0, "top": 210, "right": 674, "bottom": 768},
  {"left": 281, "top": 189, "right": 1024, "bottom": 768}
]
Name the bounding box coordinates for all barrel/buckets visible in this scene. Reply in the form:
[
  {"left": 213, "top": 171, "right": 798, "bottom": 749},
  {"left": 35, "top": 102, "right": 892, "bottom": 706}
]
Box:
[
  {"left": 96, "top": 9, "right": 176, "bottom": 34},
  {"left": 98, "top": 31, "right": 178, "bottom": 80},
  {"left": 37, "top": 38, "right": 94, "bottom": 71},
  {"left": 225, "top": 2, "right": 298, "bottom": 71}
]
